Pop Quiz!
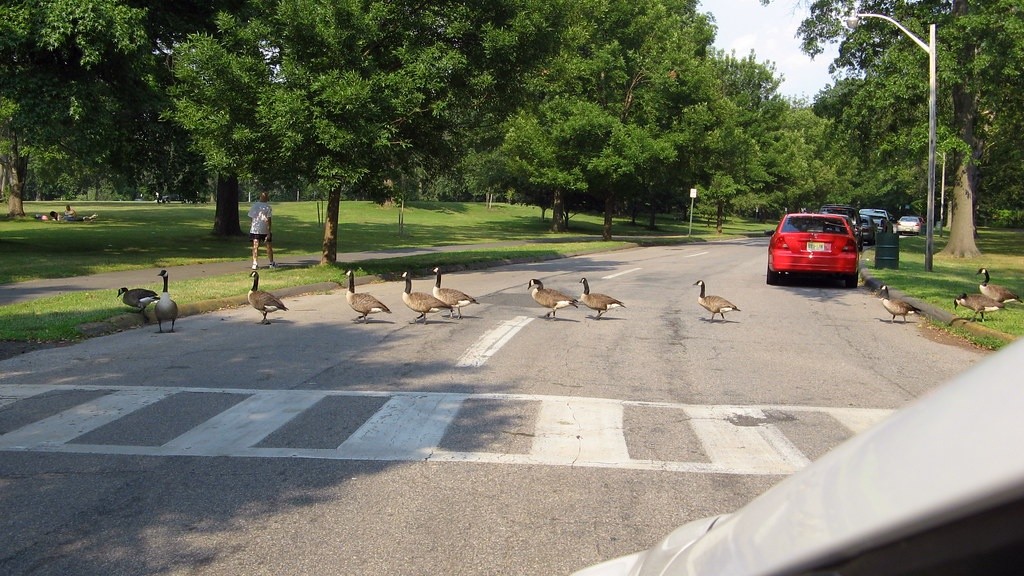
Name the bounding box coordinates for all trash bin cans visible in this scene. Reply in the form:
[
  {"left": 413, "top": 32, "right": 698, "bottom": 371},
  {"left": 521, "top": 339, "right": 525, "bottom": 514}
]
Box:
[{"left": 875, "top": 222, "right": 899, "bottom": 269}]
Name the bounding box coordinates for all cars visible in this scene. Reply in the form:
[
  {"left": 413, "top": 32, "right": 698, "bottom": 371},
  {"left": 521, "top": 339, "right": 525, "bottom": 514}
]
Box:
[
  {"left": 813, "top": 204, "right": 894, "bottom": 247},
  {"left": 763, "top": 212, "right": 863, "bottom": 288},
  {"left": 895, "top": 216, "right": 925, "bottom": 236}
]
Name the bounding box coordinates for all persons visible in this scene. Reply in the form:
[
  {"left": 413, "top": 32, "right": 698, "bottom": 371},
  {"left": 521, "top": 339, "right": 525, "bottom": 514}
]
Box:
[
  {"left": 50, "top": 205, "right": 84, "bottom": 222},
  {"left": 247, "top": 192, "right": 276, "bottom": 270},
  {"left": 155, "top": 191, "right": 170, "bottom": 204}
]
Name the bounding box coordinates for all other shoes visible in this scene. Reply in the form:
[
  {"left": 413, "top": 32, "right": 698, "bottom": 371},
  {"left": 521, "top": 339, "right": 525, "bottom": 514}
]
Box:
[
  {"left": 270, "top": 262, "right": 277, "bottom": 269},
  {"left": 252, "top": 262, "right": 258, "bottom": 269}
]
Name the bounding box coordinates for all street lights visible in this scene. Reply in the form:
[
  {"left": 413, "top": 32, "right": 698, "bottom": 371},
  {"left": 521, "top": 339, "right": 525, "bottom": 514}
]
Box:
[{"left": 845, "top": 9, "right": 936, "bottom": 274}]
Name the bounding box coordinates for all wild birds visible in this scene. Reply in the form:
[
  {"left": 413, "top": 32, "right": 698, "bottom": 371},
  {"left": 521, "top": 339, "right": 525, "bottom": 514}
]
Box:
[
  {"left": 155, "top": 270, "right": 178, "bottom": 333},
  {"left": 399, "top": 271, "right": 454, "bottom": 324},
  {"left": 954, "top": 293, "right": 1004, "bottom": 323},
  {"left": 693, "top": 279, "right": 742, "bottom": 324},
  {"left": 527, "top": 279, "right": 579, "bottom": 321},
  {"left": 247, "top": 271, "right": 290, "bottom": 325},
  {"left": 116, "top": 287, "right": 158, "bottom": 318},
  {"left": 879, "top": 284, "right": 922, "bottom": 324},
  {"left": 339, "top": 269, "right": 393, "bottom": 324},
  {"left": 580, "top": 277, "right": 628, "bottom": 321},
  {"left": 432, "top": 267, "right": 480, "bottom": 320},
  {"left": 976, "top": 267, "right": 1023, "bottom": 305}
]
